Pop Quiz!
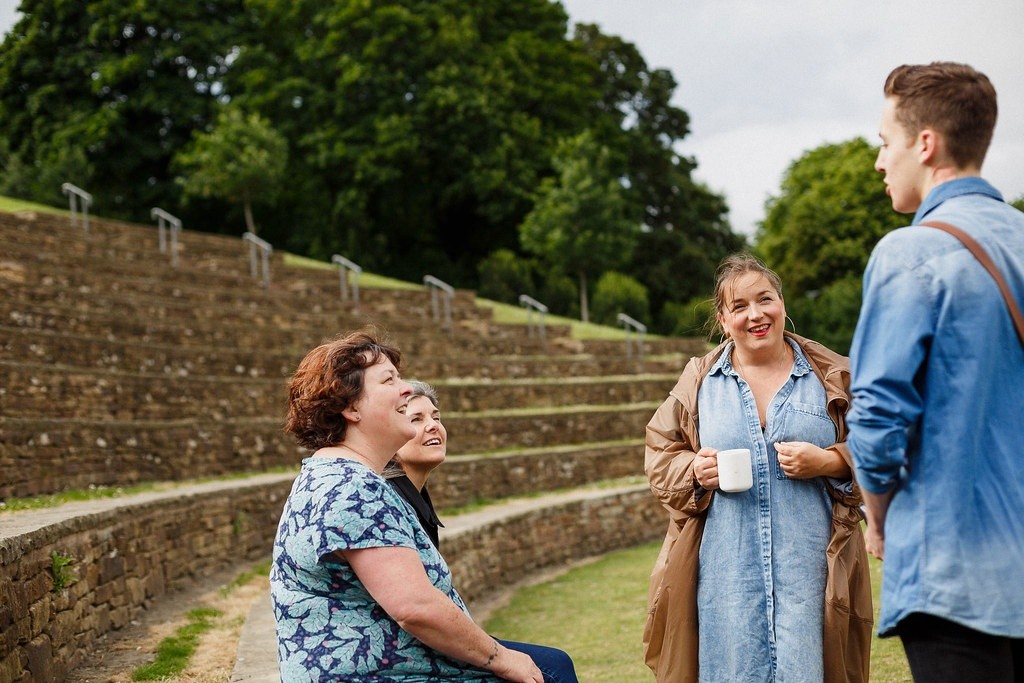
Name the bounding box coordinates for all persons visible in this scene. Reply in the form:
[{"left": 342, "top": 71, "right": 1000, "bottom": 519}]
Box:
[
  {"left": 847, "top": 58, "right": 1024, "bottom": 683},
  {"left": 381, "top": 378, "right": 579, "bottom": 683},
  {"left": 644, "top": 253, "right": 873, "bottom": 683},
  {"left": 266, "top": 322, "right": 546, "bottom": 683}
]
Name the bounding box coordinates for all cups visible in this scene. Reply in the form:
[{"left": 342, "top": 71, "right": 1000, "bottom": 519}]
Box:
[{"left": 717, "top": 449, "right": 752, "bottom": 492}]
